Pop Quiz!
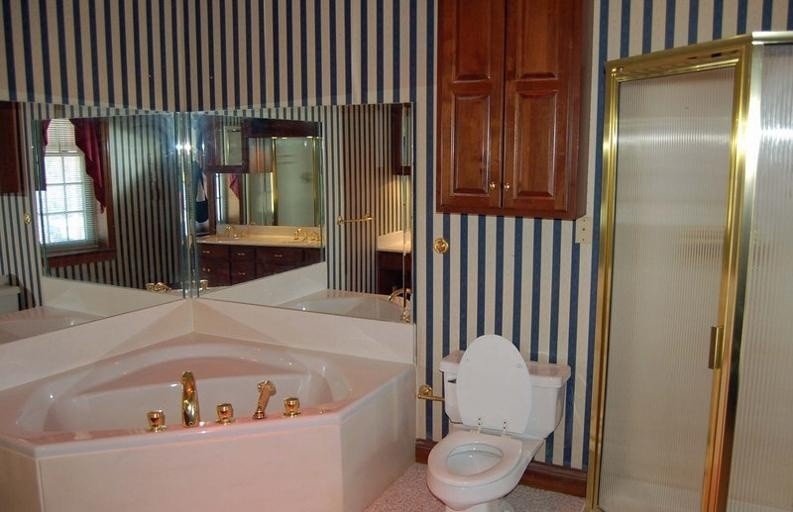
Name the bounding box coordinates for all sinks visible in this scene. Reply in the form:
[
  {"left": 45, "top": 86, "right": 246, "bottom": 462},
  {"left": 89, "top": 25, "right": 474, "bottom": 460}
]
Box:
[
  {"left": 200, "top": 234, "right": 238, "bottom": 242},
  {"left": 285, "top": 237, "right": 313, "bottom": 244}
]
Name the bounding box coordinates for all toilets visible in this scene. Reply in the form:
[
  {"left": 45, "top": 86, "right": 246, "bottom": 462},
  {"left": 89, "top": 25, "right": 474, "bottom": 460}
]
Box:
[{"left": 425, "top": 332, "right": 571, "bottom": 511}]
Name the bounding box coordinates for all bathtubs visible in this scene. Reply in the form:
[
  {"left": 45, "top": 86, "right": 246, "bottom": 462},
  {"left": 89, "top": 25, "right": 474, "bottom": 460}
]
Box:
[
  {"left": 1, "top": 306, "right": 108, "bottom": 349},
  {"left": 0, "top": 333, "right": 415, "bottom": 509},
  {"left": 278, "top": 284, "right": 412, "bottom": 322}
]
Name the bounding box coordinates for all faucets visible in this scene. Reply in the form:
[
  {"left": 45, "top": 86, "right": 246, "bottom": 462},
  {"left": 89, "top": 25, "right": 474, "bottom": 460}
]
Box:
[
  {"left": 313, "top": 230, "right": 320, "bottom": 239},
  {"left": 225, "top": 223, "right": 235, "bottom": 238},
  {"left": 147, "top": 281, "right": 175, "bottom": 294},
  {"left": 294, "top": 226, "right": 308, "bottom": 239},
  {"left": 254, "top": 377, "right": 278, "bottom": 418},
  {"left": 388, "top": 288, "right": 412, "bottom": 324},
  {"left": 177, "top": 367, "right": 202, "bottom": 426}
]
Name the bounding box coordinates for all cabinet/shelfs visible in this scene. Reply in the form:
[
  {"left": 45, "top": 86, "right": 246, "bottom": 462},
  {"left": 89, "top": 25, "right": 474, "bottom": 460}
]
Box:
[{"left": 437, "top": 0, "right": 593, "bottom": 220}]
